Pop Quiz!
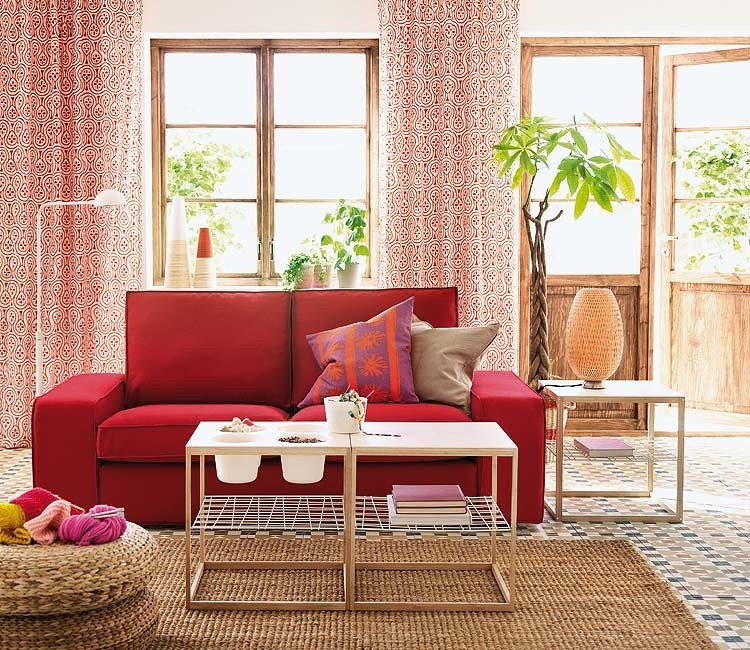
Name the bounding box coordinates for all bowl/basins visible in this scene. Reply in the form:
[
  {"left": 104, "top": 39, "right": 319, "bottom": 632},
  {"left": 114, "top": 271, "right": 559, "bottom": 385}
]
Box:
[{"left": 324, "top": 395, "right": 367, "bottom": 434}]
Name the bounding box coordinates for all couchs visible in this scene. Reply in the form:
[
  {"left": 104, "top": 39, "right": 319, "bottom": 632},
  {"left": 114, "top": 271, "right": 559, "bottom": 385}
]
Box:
[{"left": 30, "top": 284, "right": 546, "bottom": 525}]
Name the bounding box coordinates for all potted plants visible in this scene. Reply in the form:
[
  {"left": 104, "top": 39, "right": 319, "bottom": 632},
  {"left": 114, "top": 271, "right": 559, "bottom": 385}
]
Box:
[
  {"left": 487, "top": 115, "right": 640, "bottom": 462},
  {"left": 280, "top": 199, "right": 368, "bottom": 292}
]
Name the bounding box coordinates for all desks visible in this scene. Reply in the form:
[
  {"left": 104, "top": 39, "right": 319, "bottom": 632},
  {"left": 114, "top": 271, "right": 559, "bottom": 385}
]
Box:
[{"left": 539, "top": 379, "right": 684, "bottom": 523}]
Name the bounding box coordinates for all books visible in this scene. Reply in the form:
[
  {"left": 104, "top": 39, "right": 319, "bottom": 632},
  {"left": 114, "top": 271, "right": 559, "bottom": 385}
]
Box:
[
  {"left": 387, "top": 484, "right": 472, "bottom": 527},
  {"left": 573, "top": 437, "right": 634, "bottom": 457}
]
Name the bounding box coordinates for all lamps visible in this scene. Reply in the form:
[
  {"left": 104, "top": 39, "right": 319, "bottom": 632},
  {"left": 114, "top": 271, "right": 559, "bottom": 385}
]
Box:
[
  {"left": 566, "top": 289, "right": 624, "bottom": 389},
  {"left": 36, "top": 191, "right": 126, "bottom": 399}
]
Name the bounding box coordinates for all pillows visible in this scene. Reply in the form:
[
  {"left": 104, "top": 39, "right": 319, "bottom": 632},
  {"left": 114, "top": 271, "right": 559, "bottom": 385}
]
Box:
[
  {"left": 297, "top": 296, "right": 420, "bottom": 407},
  {"left": 411, "top": 311, "right": 501, "bottom": 415}
]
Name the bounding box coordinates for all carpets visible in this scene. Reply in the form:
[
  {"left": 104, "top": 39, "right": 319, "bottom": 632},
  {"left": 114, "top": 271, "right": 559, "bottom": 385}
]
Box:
[{"left": 153, "top": 535, "right": 721, "bottom": 650}]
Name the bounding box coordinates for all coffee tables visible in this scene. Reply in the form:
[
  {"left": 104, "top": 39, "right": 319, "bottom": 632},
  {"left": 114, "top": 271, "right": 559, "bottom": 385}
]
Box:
[{"left": 187, "top": 422, "right": 518, "bottom": 612}]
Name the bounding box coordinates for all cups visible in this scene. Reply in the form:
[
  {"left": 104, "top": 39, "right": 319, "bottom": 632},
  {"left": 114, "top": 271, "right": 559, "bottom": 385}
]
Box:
[{"left": 165, "top": 196, "right": 216, "bottom": 288}]
